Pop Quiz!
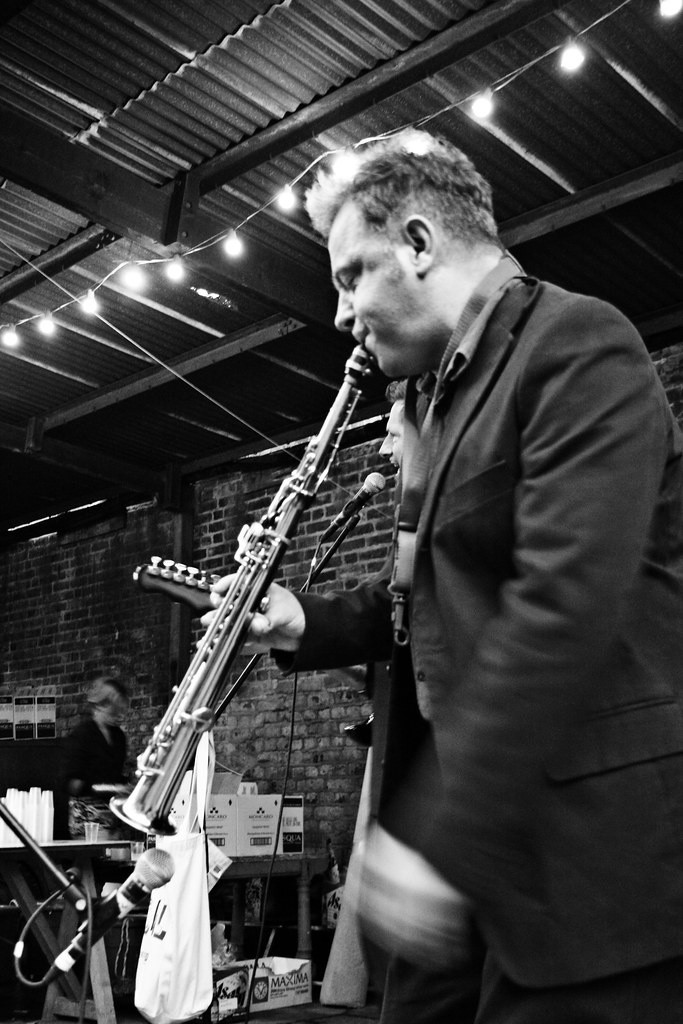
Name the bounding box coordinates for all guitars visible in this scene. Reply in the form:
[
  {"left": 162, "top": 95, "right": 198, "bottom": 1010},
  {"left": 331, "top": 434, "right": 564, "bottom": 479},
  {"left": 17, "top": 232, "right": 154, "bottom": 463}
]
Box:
[{"left": 127, "top": 555, "right": 378, "bottom": 749}]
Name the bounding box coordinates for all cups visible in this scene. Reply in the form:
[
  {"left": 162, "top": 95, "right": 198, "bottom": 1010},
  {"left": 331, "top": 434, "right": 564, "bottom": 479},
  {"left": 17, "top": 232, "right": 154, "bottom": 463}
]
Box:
[
  {"left": 0, "top": 786, "right": 55, "bottom": 847},
  {"left": 84, "top": 822, "right": 99, "bottom": 843}
]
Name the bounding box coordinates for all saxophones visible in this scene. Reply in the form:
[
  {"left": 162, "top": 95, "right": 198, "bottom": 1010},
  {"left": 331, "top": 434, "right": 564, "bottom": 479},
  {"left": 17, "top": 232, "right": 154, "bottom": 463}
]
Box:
[{"left": 106, "top": 344, "right": 377, "bottom": 839}]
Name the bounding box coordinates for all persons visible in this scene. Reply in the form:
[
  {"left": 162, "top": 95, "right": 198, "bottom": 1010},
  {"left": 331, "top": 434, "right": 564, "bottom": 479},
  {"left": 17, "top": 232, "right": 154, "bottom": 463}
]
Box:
[
  {"left": 48, "top": 677, "right": 132, "bottom": 838},
  {"left": 198, "top": 133, "right": 682, "bottom": 1024}
]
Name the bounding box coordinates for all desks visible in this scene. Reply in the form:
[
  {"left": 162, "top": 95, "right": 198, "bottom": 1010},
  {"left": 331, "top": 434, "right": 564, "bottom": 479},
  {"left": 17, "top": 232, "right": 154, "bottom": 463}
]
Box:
[
  {"left": 219, "top": 853, "right": 333, "bottom": 962},
  {"left": 0, "top": 840, "right": 131, "bottom": 1024}
]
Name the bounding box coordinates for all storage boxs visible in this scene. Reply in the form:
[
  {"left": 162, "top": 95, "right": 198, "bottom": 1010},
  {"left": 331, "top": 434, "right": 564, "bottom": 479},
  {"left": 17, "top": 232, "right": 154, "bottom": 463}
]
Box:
[
  {"left": 169, "top": 771, "right": 303, "bottom": 857},
  {"left": 0, "top": 684, "right": 58, "bottom": 741},
  {"left": 212, "top": 954, "right": 313, "bottom": 1023}
]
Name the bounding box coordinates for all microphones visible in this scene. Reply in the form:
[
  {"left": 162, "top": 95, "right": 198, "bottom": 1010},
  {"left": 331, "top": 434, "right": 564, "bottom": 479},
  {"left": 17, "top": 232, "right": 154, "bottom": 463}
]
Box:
[
  {"left": 43, "top": 848, "right": 176, "bottom": 985},
  {"left": 319, "top": 472, "right": 385, "bottom": 543}
]
